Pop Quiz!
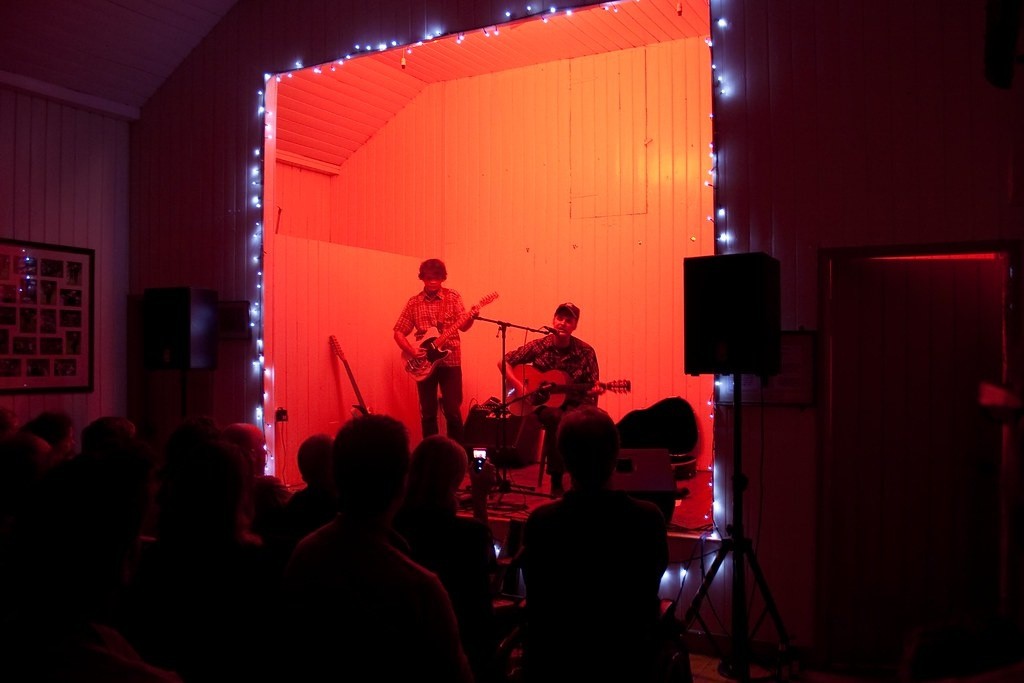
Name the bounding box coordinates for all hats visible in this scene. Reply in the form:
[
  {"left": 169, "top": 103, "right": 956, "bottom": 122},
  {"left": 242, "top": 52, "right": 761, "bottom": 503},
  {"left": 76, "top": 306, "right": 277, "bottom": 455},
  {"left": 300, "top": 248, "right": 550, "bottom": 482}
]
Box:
[{"left": 557, "top": 303, "right": 580, "bottom": 323}]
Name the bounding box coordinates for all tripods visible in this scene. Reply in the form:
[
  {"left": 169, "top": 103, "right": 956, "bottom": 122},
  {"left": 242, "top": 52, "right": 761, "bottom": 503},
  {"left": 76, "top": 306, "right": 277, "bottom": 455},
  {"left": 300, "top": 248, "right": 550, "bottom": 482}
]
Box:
[
  {"left": 465, "top": 313, "right": 560, "bottom": 511},
  {"left": 672, "top": 370, "right": 797, "bottom": 683}
]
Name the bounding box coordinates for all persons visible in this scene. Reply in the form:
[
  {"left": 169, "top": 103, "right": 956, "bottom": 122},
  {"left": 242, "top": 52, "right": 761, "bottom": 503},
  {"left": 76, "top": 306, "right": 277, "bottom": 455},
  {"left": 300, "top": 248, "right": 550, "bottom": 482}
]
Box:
[
  {"left": 522, "top": 406, "right": 676, "bottom": 683},
  {"left": 392, "top": 258, "right": 480, "bottom": 446},
  {"left": 498, "top": 303, "right": 600, "bottom": 499},
  {"left": 0, "top": 412, "right": 515, "bottom": 683}
]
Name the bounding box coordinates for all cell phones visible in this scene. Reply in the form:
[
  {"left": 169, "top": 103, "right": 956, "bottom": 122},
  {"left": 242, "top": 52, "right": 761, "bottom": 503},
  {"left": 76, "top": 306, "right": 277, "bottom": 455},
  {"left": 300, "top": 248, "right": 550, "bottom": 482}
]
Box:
[{"left": 471, "top": 448, "right": 487, "bottom": 472}]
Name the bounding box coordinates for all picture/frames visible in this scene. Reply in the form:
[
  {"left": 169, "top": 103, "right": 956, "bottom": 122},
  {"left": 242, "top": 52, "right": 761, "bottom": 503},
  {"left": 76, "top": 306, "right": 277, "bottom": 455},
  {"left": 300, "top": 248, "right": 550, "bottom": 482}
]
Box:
[
  {"left": 215, "top": 300, "right": 252, "bottom": 341},
  {"left": 0, "top": 238, "right": 96, "bottom": 394}
]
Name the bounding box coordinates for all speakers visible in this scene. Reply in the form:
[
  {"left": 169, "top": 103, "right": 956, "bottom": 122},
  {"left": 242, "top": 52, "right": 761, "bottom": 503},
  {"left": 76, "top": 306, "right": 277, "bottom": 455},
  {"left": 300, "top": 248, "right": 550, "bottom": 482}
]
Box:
[
  {"left": 141, "top": 287, "right": 218, "bottom": 373},
  {"left": 683, "top": 251, "right": 782, "bottom": 379},
  {"left": 461, "top": 405, "right": 541, "bottom": 469}
]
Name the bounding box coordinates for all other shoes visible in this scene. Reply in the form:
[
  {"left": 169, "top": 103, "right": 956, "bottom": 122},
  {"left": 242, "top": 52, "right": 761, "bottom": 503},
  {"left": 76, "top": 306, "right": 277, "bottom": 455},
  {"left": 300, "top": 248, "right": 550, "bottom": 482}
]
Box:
[
  {"left": 550, "top": 486, "right": 562, "bottom": 499},
  {"left": 458, "top": 492, "right": 473, "bottom": 506}
]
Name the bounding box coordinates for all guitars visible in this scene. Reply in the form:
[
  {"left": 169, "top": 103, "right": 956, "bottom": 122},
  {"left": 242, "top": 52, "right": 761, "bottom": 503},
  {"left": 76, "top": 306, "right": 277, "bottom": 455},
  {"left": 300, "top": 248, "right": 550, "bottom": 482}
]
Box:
[
  {"left": 505, "top": 364, "right": 631, "bottom": 416},
  {"left": 400, "top": 292, "right": 499, "bottom": 383},
  {"left": 328, "top": 335, "right": 373, "bottom": 417}
]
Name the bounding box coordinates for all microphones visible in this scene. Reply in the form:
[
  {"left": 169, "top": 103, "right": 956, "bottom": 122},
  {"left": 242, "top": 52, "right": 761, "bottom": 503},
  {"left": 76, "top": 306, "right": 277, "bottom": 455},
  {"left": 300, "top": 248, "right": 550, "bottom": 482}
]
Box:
[{"left": 543, "top": 326, "right": 560, "bottom": 336}]
